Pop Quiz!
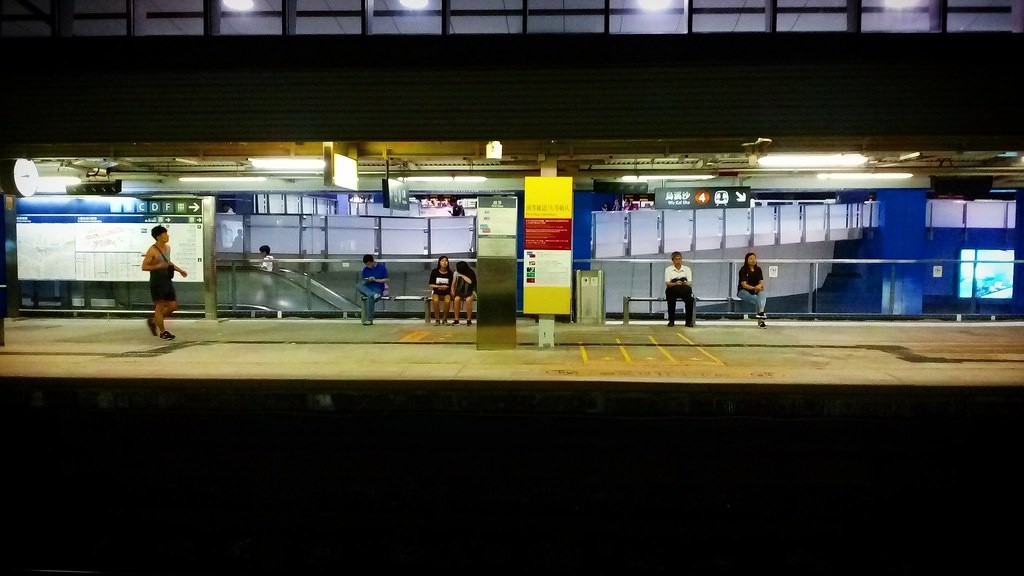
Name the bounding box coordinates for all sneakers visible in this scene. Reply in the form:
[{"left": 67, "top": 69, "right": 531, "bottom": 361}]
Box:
[
  {"left": 147, "top": 318, "right": 157, "bottom": 336},
  {"left": 159, "top": 331, "right": 175, "bottom": 341}
]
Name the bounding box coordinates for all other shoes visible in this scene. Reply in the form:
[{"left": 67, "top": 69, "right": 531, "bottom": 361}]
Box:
[
  {"left": 686, "top": 321, "right": 693, "bottom": 327},
  {"left": 363, "top": 320, "right": 372, "bottom": 325},
  {"left": 434, "top": 320, "right": 439, "bottom": 326},
  {"left": 668, "top": 321, "right": 674, "bottom": 326},
  {"left": 756, "top": 312, "right": 767, "bottom": 318},
  {"left": 452, "top": 320, "right": 459, "bottom": 326},
  {"left": 758, "top": 322, "right": 767, "bottom": 329},
  {"left": 373, "top": 293, "right": 381, "bottom": 302},
  {"left": 467, "top": 320, "right": 472, "bottom": 326},
  {"left": 441, "top": 320, "right": 447, "bottom": 325}
]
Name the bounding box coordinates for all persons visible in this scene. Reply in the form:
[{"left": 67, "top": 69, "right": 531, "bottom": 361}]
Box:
[
  {"left": 356, "top": 254, "right": 390, "bottom": 325},
  {"left": 259, "top": 245, "right": 274, "bottom": 271},
  {"left": 602, "top": 203, "right": 607, "bottom": 211},
  {"left": 736, "top": 251, "right": 767, "bottom": 328},
  {"left": 141, "top": 225, "right": 187, "bottom": 339},
  {"left": 622, "top": 197, "right": 631, "bottom": 211},
  {"left": 222, "top": 203, "right": 234, "bottom": 213},
  {"left": 664, "top": 252, "right": 694, "bottom": 327},
  {"left": 612, "top": 199, "right": 622, "bottom": 211},
  {"left": 447, "top": 198, "right": 466, "bottom": 217},
  {"left": 450, "top": 261, "right": 477, "bottom": 326},
  {"left": 429, "top": 256, "right": 452, "bottom": 326}
]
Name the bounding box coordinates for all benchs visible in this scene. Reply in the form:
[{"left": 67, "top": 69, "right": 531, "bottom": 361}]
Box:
[
  {"left": 623, "top": 296, "right": 764, "bottom": 327},
  {"left": 360, "top": 296, "right": 477, "bottom": 325}
]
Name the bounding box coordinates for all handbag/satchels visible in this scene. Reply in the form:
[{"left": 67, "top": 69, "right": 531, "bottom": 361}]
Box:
[
  {"left": 669, "top": 277, "right": 691, "bottom": 301},
  {"left": 168, "top": 264, "right": 174, "bottom": 279}
]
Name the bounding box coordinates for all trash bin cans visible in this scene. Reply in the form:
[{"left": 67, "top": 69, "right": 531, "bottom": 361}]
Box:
[{"left": 575, "top": 269, "right": 607, "bottom": 324}]
